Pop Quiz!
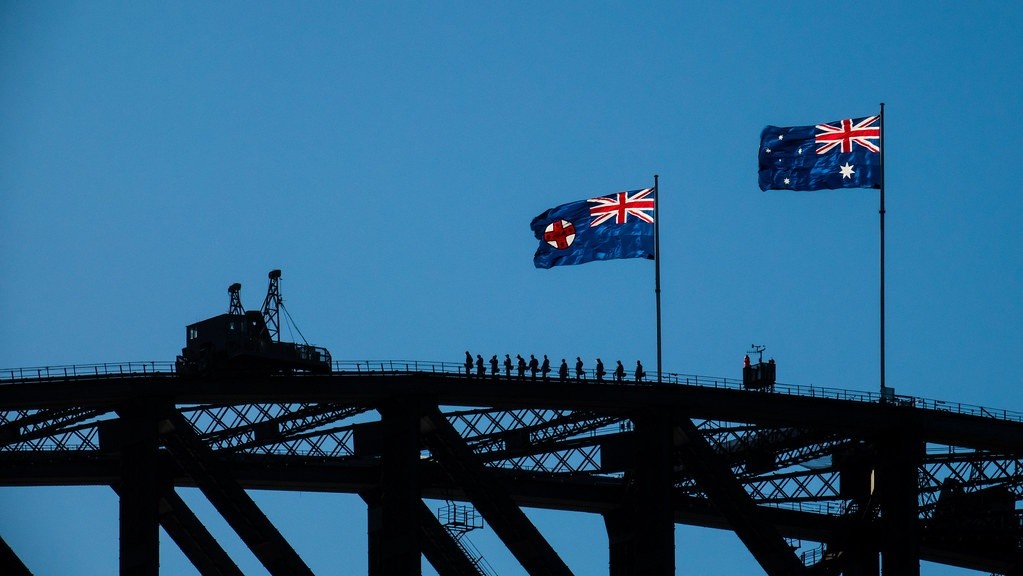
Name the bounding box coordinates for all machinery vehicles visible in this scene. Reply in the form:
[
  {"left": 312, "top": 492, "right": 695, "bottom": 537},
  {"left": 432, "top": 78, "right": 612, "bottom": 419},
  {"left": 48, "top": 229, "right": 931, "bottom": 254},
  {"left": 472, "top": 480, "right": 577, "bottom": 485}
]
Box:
[{"left": 174, "top": 310, "right": 333, "bottom": 373}]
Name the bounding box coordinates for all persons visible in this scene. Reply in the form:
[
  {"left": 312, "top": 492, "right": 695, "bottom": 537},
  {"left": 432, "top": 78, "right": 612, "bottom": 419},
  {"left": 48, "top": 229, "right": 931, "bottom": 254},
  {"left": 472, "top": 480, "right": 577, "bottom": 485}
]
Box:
[
  {"left": 576, "top": 357, "right": 583, "bottom": 379},
  {"left": 515, "top": 354, "right": 526, "bottom": 377},
  {"left": 596, "top": 358, "right": 604, "bottom": 379},
  {"left": 559, "top": 358, "right": 567, "bottom": 379},
  {"left": 490, "top": 354, "right": 500, "bottom": 376},
  {"left": 635, "top": 360, "right": 644, "bottom": 381},
  {"left": 477, "top": 355, "right": 485, "bottom": 374},
  {"left": 615, "top": 360, "right": 623, "bottom": 380},
  {"left": 464, "top": 350, "right": 473, "bottom": 373},
  {"left": 541, "top": 354, "right": 550, "bottom": 378},
  {"left": 528, "top": 355, "right": 539, "bottom": 376},
  {"left": 504, "top": 354, "right": 512, "bottom": 376}
]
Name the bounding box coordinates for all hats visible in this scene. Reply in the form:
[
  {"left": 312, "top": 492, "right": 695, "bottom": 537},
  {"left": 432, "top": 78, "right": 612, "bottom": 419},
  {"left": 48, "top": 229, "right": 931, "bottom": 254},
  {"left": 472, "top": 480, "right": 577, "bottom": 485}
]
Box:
[
  {"left": 530, "top": 355, "right": 534, "bottom": 357},
  {"left": 616, "top": 360, "right": 621, "bottom": 362},
  {"left": 504, "top": 353, "right": 510, "bottom": 356},
  {"left": 515, "top": 353, "right": 521, "bottom": 358},
  {"left": 576, "top": 357, "right": 580, "bottom": 359}
]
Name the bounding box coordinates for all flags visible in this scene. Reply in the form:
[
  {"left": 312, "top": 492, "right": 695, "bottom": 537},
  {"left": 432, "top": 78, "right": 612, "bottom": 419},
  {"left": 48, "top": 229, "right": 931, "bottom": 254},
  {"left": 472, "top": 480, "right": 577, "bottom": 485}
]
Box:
[
  {"left": 757, "top": 113, "right": 881, "bottom": 195},
  {"left": 530, "top": 188, "right": 655, "bottom": 269}
]
